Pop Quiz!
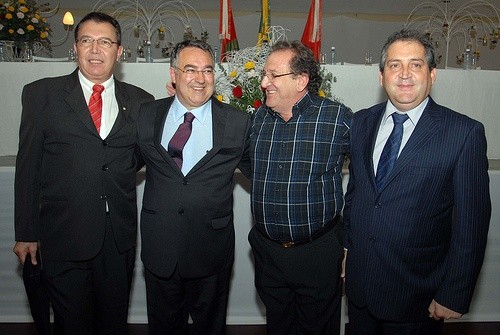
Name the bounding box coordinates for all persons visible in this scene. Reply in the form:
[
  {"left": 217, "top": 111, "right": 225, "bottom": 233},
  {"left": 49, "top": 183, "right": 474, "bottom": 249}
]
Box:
[
  {"left": 166, "top": 40, "right": 353, "bottom": 335},
  {"left": 12, "top": 13, "right": 156, "bottom": 335},
  {"left": 341, "top": 32, "right": 492, "bottom": 335},
  {"left": 136, "top": 39, "right": 252, "bottom": 335}
]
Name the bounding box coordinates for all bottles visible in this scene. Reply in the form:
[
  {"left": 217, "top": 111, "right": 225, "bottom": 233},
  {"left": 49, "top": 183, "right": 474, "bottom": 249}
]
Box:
[
  {"left": 260, "top": 40, "right": 271, "bottom": 62},
  {"left": 213, "top": 45, "right": 220, "bottom": 63},
  {"left": 463, "top": 48, "right": 472, "bottom": 70},
  {"left": 329, "top": 46, "right": 336, "bottom": 65},
  {"left": 0, "top": 43, "right": 5, "bottom": 62},
  {"left": 145, "top": 41, "right": 153, "bottom": 63}
]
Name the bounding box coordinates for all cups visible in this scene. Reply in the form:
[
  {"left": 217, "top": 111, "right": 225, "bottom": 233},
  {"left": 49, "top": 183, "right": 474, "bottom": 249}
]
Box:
[
  {"left": 68, "top": 49, "right": 76, "bottom": 62},
  {"left": 319, "top": 53, "right": 326, "bottom": 65},
  {"left": 24, "top": 48, "right": 33, "bottom": 63},
  {"left": 364, "top": 49, "right": 373, "bottom": 66},
  {"left": 120, "top": 48, "right": 128, "bottom": 63}
]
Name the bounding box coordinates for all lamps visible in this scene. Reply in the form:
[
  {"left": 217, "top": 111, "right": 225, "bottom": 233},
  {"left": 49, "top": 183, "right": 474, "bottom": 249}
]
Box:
[{"left": 50, "top": 11, "right": 74, "bottom": 43}]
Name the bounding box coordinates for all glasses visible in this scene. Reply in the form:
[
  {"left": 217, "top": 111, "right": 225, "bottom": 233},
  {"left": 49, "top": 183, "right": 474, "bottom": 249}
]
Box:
[
  {"left": 74, "top": 36, "right": 118, "bottom": 48},
  {"left": 260, "top": 70, "right": 298, "bottom": 82},
  {"left": 174, "top": 65, "right": 216, "bottom": 77}
]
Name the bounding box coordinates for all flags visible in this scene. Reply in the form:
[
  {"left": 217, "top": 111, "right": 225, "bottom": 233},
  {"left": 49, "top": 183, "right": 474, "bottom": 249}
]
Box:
[
  {"left": 301, "top": 0, "right": 322, "bottom": 60},
  {"left": 219, "top": 0, "right": 239, "bottom": 62},
  {"left": 259, "top": 0, "right": 272, "bottom": 44}
]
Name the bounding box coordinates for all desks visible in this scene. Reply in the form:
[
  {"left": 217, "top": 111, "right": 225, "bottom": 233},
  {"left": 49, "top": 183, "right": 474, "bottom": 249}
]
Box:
[
  {"left": 0, "top": 167, "right": 499, "bottom": 325},
  {"left": 0, "top": 61, "right": 500, "bottom": 161}
]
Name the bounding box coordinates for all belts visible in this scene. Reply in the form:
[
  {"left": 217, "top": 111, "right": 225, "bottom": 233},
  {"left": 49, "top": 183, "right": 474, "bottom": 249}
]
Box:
[{"left": 255, "top": 214, "right": 340, "bottom": 248}]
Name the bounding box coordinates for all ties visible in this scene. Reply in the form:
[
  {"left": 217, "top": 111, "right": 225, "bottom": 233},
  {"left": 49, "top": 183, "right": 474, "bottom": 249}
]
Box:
[
  {"left": 88, "top": 85, "right": 105, "bottom": 135},
  {"left": 168, "top": 113, "right": 195, "bottom": 170},
  {"left": 375, "top": 114, "right": 409, "bottom": 192}
]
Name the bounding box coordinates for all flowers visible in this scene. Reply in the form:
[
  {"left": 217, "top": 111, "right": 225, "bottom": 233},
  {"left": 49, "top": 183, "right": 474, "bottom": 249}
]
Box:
[
  {"left": 0, "top": 0, "right": 53, "bottom": 52},
  {"left": 212, "top": 61, "right": 337, "bottom": 113}
]
